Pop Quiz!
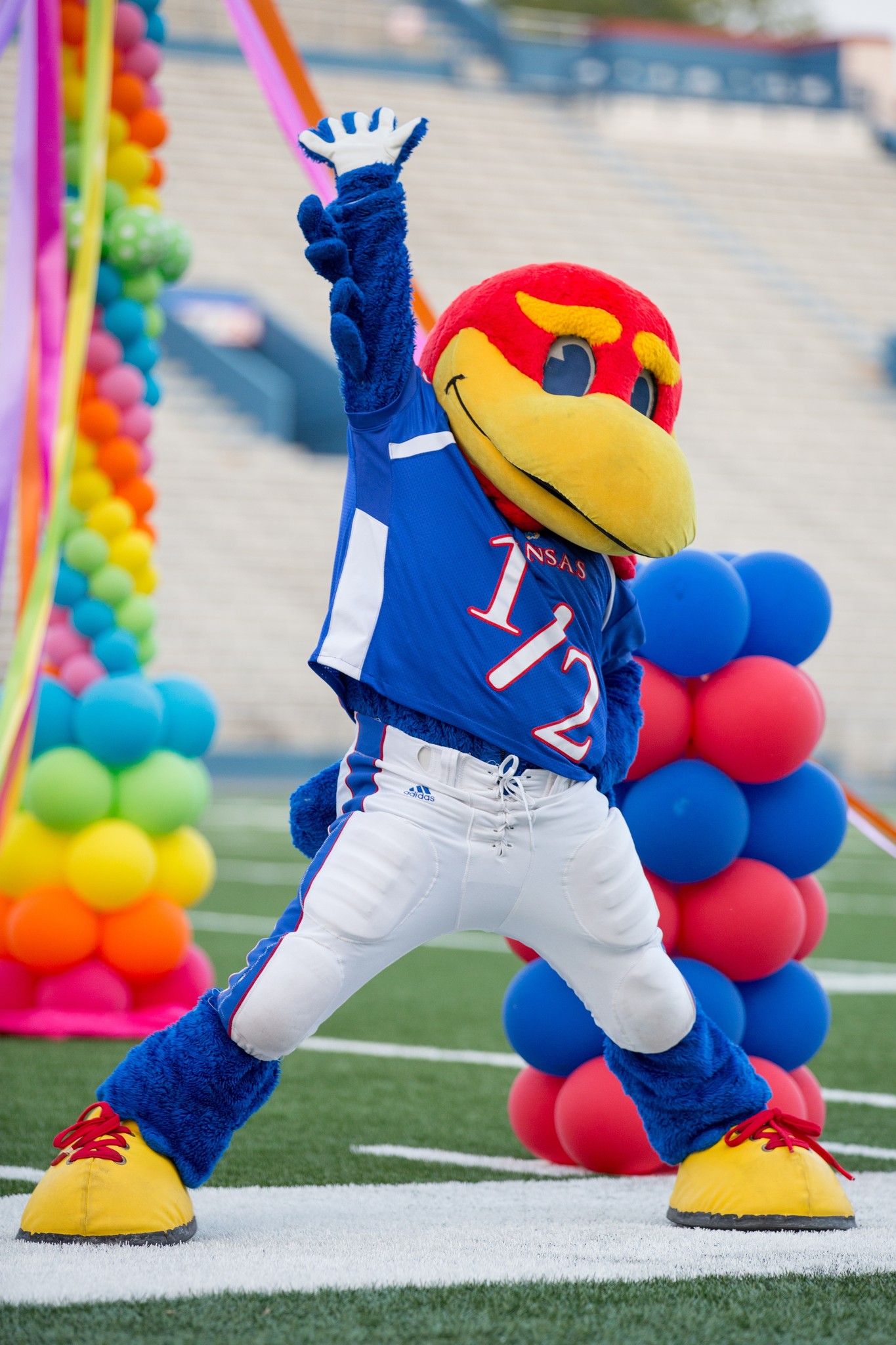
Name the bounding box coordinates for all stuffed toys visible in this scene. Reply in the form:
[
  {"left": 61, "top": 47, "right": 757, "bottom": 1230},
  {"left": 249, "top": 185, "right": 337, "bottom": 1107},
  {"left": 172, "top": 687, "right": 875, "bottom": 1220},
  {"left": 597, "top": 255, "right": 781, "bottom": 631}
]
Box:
[{"left": 15, "top": 102, "right": 859, "bottom": 1248}]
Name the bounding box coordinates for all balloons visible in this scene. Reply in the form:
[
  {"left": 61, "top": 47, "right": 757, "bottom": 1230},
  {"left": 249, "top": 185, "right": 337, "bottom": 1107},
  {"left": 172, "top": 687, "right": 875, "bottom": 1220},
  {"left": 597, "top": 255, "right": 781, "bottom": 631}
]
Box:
[
  {"left": 0, "top": 19, "right": 217, "bottom": 1042},
  {"left": 494, "top": 536, "right": 853, "bottom": 1180}
]
